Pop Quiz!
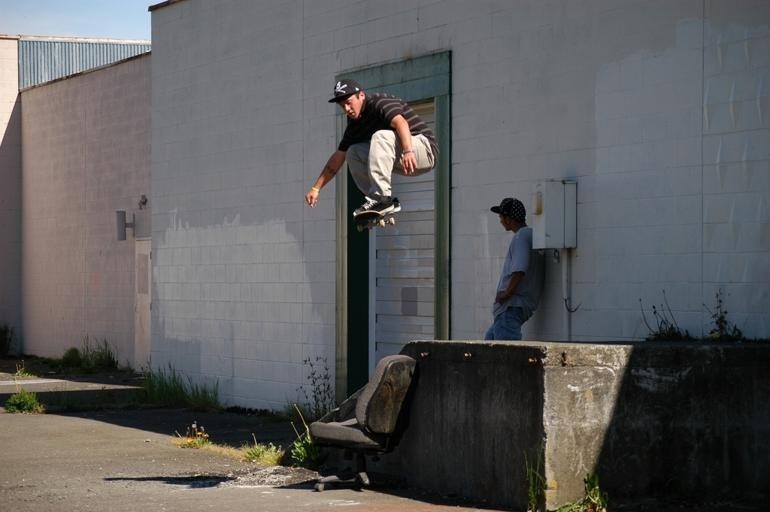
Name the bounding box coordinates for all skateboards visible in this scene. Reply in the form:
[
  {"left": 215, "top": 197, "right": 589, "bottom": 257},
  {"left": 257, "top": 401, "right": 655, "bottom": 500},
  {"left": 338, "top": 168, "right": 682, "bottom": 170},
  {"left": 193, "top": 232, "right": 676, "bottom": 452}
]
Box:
[{"left": 353, "top": 211, "right": 395, "bottom": 232}]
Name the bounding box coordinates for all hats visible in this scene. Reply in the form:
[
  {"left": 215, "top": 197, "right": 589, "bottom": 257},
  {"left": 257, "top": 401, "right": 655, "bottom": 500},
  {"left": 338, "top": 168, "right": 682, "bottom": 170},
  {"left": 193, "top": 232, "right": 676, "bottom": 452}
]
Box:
[
  {"left": 328, "top": 79, "right": 363, "bottom": 102},
  {"left": 490, "top": 198, "right": 525, "bottom": 221}
]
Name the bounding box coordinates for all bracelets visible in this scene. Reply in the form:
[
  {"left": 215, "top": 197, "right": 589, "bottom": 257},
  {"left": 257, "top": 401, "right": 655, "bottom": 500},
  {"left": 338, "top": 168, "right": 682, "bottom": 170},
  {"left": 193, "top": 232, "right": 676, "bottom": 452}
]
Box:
[
  {"left": 403, "top": 150, "right": 416, "bottom": 154},
  {"left": 309, "top": 187, "right": 320, "bottom": 193}
]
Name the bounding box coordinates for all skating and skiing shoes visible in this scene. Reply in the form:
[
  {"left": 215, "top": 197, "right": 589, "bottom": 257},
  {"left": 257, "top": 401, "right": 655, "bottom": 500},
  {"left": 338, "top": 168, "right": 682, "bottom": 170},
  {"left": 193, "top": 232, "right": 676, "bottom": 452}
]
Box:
[{"left": 353, "top": 191, "right": 401, "bottom": 221}]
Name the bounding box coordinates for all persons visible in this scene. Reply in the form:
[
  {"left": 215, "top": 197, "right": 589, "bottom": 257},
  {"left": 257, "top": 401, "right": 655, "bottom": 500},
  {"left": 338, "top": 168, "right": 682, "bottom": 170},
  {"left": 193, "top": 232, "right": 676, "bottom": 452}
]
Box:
[
  {"left": 305, "top": 80, "right": 440, "bottom": 216},
  {"left": 484, "top": 198, "right": 543, "bottom": 340}
]
